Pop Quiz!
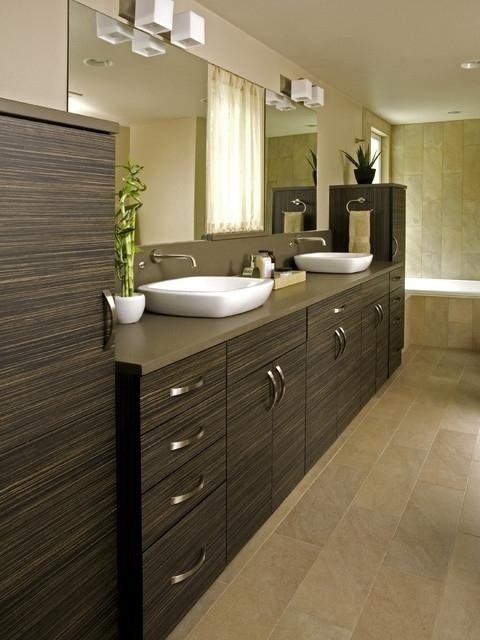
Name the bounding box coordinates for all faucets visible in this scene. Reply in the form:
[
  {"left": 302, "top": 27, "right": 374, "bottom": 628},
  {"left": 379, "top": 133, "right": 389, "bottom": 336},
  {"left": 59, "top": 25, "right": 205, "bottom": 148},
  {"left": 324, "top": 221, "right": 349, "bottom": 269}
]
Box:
[
  {"left": 286, "top": 235, "right": 328, "bottom": 248},
  {"left": 148, "top": 247, "right": 200, "bottom": 270}
]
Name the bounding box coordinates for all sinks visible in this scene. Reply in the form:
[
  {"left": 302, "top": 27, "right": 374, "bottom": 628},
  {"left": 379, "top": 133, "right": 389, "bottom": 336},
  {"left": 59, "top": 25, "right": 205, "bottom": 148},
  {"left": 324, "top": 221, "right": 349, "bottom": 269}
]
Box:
[
  {"left": 137, "top": 274, "right": 279, "bottom": 317},
  {"left": 292, "top": 249, "right": 374, "bottom": 275}
]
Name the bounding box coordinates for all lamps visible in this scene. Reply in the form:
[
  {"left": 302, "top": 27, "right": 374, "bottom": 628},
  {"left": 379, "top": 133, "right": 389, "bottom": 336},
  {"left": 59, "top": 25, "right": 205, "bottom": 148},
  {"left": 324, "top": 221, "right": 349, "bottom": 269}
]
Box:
[
  {"left": 266, "top": 90, "right": 282, "bottom": 106},
  {"left": 304, "top": 87, "right": 325, "bottom": 109},
  {"left": 276, "top": 97, "right": 296, "bottom": 111},
  {"left": 131, "top": 30, "right": 167, "bottom": 58},
  {"left": 170, "top": 10, "right": 206, "bottom": 49},
  {"left": 134, "top": 1, "right": 175, "bottom": 35},
  {"left": 290, "top": 79, "right": 313, "bottom": 102},
  {"left": 96, "top": 13, "right": 134, "bottom": 44}
]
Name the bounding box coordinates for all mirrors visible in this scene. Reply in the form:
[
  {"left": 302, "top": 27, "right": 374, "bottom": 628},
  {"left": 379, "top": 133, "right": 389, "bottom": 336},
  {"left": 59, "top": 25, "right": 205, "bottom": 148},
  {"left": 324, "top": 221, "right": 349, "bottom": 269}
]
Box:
[{"left": 67, "top": 1, "right": 317, "bottom": 246}]
